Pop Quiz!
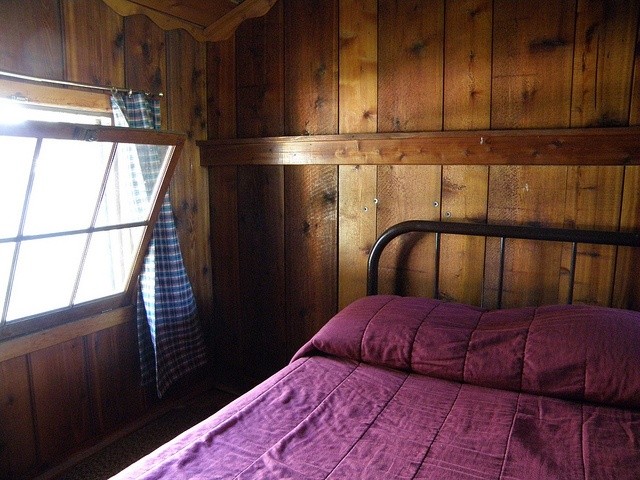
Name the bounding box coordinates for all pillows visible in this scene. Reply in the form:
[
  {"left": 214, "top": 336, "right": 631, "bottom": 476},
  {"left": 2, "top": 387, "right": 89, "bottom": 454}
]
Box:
[{"left": 290, "top": 295, "right": 640, "bottom": 412}]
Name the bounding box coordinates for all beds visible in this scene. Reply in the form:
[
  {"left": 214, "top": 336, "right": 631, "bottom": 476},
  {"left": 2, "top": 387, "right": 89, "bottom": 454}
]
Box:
[{"left": 108, "top": 221, "right": 640, "bottom": 480}]
